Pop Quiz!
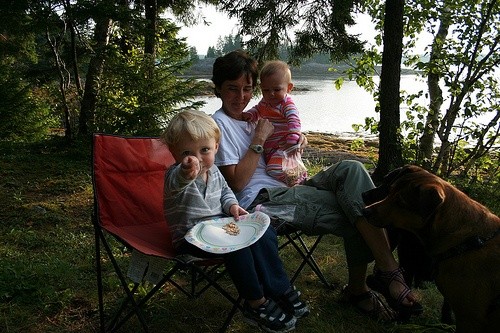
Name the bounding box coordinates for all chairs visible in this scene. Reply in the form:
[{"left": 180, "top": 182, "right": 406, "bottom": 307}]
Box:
[
  {"left": 87, "top": 132, "right": 273, "bottom": 333},
  {"left": 265, "top": 214, "right": 339, "bottom": 299}
]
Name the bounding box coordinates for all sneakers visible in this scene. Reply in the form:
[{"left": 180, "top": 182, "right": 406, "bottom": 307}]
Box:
[
  {"left": 277, "top": 291, "right": 310, "bottom": 318},
  {"left": 242, "top": 297, "right": 298, "bottom": 333}
]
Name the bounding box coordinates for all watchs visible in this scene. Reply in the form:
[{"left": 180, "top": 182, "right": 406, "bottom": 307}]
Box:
[{"left": 248, "top": 144, "right": 264, "bottom": 154}]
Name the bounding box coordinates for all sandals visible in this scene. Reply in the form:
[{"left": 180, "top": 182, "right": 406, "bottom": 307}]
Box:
[
  {"left": 366, "top": 264, "right": 423, "bottom": 314},
  {"left": 338, "top": 284, "right": 400, "bottom": 325}
]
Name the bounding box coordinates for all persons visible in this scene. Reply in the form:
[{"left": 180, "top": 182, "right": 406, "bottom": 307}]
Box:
[
  {"left": 228, "top": 60, "right": 308, "bottom": 187},
  {"left": 161, "top": 108, "right": 310, "bottom": 333},
  {"left": 202, "top": 50, "right": 422, "bottom": 326}
]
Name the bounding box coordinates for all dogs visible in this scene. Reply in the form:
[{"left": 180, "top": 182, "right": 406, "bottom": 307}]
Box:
[
  {"left": 359, "top": 164, "right": 454, "bottom": 326},
  {"left": 362, "top": 169, "right": 500, "bottom": 333}
]
